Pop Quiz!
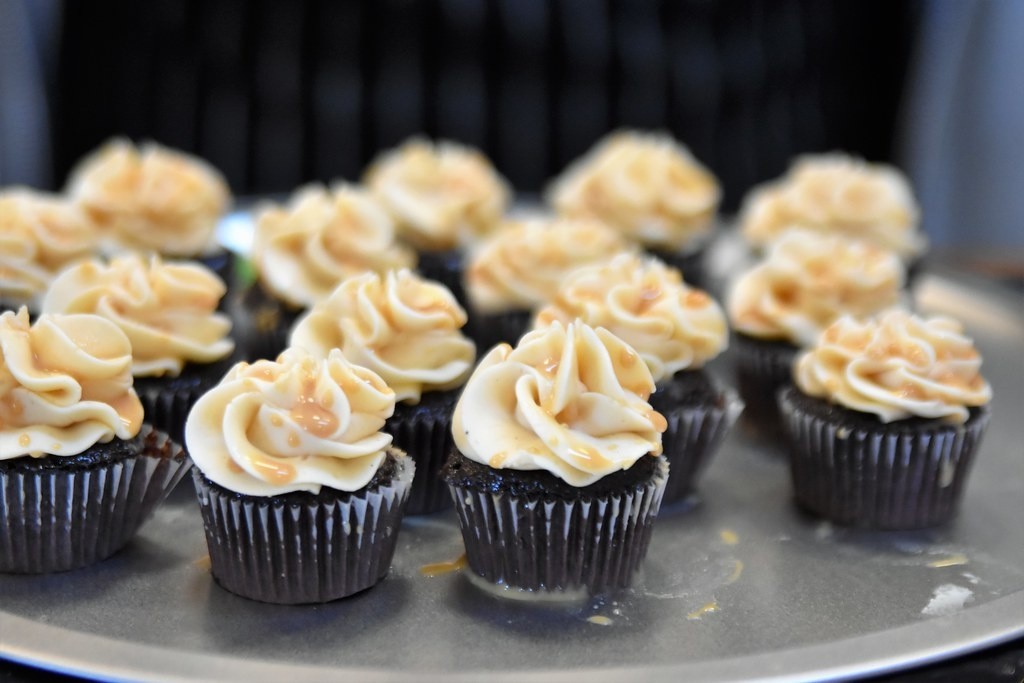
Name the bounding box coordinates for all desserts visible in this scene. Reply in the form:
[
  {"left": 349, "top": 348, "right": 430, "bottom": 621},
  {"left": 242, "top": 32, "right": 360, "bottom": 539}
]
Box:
[{"left": 0, "top": 125, "right": 993, "bottom": 600}]
